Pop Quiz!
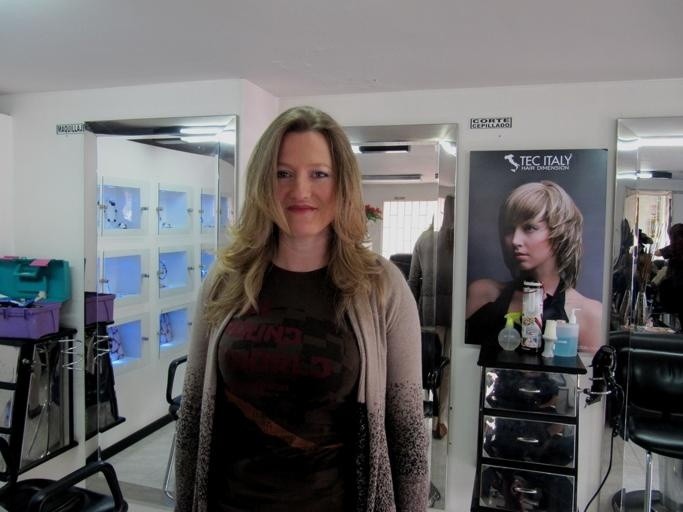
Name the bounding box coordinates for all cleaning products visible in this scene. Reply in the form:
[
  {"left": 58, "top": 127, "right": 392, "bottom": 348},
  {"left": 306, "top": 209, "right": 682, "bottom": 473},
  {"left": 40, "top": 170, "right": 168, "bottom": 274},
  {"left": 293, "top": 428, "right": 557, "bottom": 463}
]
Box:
[{"left": 498, "top": 311, "right": 522, "bottom": 352}]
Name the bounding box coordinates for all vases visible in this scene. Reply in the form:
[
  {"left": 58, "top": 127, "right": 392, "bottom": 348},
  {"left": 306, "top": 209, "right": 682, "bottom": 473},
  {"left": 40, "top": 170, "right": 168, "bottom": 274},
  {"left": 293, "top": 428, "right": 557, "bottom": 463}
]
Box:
[{"left": 362, "top": 232, "right": 372, "bottom": 250}]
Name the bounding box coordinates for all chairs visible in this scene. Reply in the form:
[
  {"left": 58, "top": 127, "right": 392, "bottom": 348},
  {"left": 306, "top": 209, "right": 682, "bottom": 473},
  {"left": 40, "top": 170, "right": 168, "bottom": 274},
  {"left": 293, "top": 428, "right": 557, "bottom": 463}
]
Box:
[
  {"left": 0, "top": 437, "right": 129, "bottom": 512},
  {"left": 609, "top": 331, "right": 683, "bottom": 512},
  {"left": 419, "top": 329, "right": 450, "bottom": 440},
  {"left": 162, "top": 355, "right": 188, "bottom": 502}
]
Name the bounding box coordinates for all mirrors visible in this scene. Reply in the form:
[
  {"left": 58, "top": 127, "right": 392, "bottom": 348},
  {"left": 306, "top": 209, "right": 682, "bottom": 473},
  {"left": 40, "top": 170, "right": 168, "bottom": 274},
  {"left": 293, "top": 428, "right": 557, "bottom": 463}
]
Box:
[
  {"left": 596, "top": 114, "right": 683, "bottom": 512},
  {"left": 82, "top": 115, "right": 237, "bottom": 508},
  {"left": 339, "top": 124, "right": 458, "bottom": 512}
]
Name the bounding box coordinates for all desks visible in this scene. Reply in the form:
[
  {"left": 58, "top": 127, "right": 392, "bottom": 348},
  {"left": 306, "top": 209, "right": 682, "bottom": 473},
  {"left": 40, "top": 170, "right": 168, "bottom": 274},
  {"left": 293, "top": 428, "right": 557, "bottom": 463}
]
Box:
[
  {"left": 85, "top": 320, "right": 125, "bottom": 440},
  {"left": 0, "top": 328, "right": 77, "bottom": 484}
]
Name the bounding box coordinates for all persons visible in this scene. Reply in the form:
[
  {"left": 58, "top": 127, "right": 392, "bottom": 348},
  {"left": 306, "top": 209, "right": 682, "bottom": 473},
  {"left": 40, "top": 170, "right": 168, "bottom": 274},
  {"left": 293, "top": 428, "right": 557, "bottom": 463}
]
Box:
[
  {"left": 465, "top": 181, "right": 603, "bottom": 355},
  {"left": 174, "top": 105, "right": 431, "bottom": 511},
  {"left": 406, "top": 223, "right": 449, "bottom": 440},
  {"left": 654, "top": 223, "right": 683, "bottom": 333}
]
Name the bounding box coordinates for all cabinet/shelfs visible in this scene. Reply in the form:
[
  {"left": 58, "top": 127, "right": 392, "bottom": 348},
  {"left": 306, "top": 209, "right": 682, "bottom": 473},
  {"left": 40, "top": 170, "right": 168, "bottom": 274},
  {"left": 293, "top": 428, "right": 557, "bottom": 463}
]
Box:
[
  {"left": 97, "top": 184, "right": 228, "bottom": 369},
  {"left": 469, "top": 341, "right": 587, "bottom": 512}
]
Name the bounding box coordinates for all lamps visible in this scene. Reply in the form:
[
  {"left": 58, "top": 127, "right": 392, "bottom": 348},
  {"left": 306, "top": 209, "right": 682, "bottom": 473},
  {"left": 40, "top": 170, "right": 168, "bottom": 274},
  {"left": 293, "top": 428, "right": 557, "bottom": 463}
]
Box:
[
  {"left": 360, "top": 147, "right": 409, "bottom": 153},
  {"left": 640, "top": 172, "right": 672, "bottom": 179}
]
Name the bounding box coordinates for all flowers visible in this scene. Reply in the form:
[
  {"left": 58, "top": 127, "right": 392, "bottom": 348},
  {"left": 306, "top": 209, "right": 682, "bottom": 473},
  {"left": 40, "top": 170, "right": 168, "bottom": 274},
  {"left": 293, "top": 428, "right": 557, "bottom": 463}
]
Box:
[{"left": 364, "top": 205, "right": 383, "bottom": 221}]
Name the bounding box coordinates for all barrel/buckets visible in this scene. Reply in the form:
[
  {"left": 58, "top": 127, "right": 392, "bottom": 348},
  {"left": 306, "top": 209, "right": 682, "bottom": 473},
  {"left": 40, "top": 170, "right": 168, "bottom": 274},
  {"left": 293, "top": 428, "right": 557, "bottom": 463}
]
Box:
[{"left": 555, "top": 308, "right": 582, "bottom": 358}]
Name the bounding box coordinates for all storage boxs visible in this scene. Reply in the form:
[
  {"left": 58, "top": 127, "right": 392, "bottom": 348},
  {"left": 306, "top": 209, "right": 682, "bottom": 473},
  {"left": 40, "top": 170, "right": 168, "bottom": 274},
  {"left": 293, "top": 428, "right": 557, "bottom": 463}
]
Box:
[
  {"left": 85, "top": 291, "right": 116, "bottom": 325},
  {"left": 0, "top": 256, "right": 71, "bottom": 339}
]
